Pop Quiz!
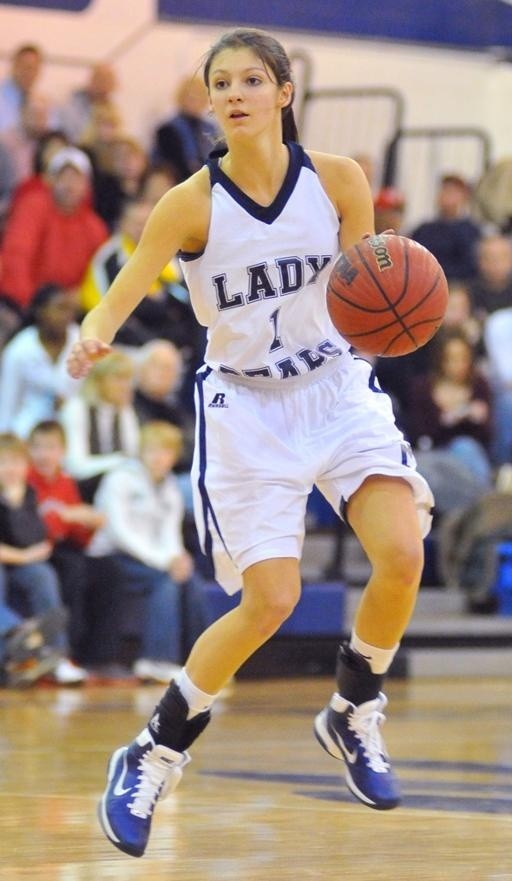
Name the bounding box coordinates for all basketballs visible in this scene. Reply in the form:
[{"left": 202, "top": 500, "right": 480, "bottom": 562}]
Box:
[{"left": 326, "top": 234, "right": 449, "bottom": 359}]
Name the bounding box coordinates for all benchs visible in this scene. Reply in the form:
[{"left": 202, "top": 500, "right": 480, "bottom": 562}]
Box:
[{"left": 169, "top": 484, "right": 510, "bottom": 681}]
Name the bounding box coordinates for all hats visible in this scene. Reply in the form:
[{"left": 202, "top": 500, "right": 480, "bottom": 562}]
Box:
[{"left": 46, "top": 146, "right": 90, "bottom": 179}]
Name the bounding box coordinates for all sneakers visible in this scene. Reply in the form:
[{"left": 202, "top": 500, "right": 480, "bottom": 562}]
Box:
[
  {"left": 4, "top": 602, "right": 86, "bottom": 687},
  {"left": 314, "top": 690, "right": 401, "bottom": 809},
  {"left": 97, "top": 728, "right": 192, "bottom": 860}
]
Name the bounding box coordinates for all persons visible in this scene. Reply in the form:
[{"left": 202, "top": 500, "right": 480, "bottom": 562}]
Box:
[{"left": 68, "top": 29, "right": 434, "bottom": 858}]
